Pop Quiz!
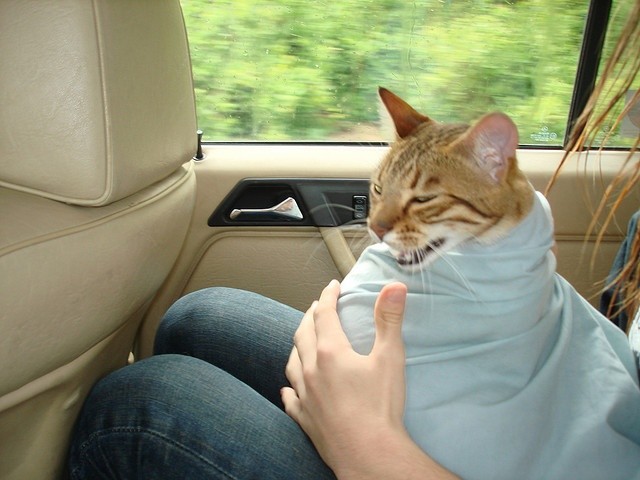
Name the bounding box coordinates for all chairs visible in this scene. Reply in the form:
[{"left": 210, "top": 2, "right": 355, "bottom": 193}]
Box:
[{"left": 0, "top": 1, "right": 198, "bottom": 480}]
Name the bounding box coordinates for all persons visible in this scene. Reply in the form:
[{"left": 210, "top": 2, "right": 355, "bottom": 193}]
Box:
[{"left": 62, "top": 0, "right": 640, "bottom": 480}]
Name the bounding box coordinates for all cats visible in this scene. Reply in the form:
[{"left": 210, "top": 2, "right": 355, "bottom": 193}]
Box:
[{"left": 334, "top": 85, "right": 560, "bottom": 480}]
